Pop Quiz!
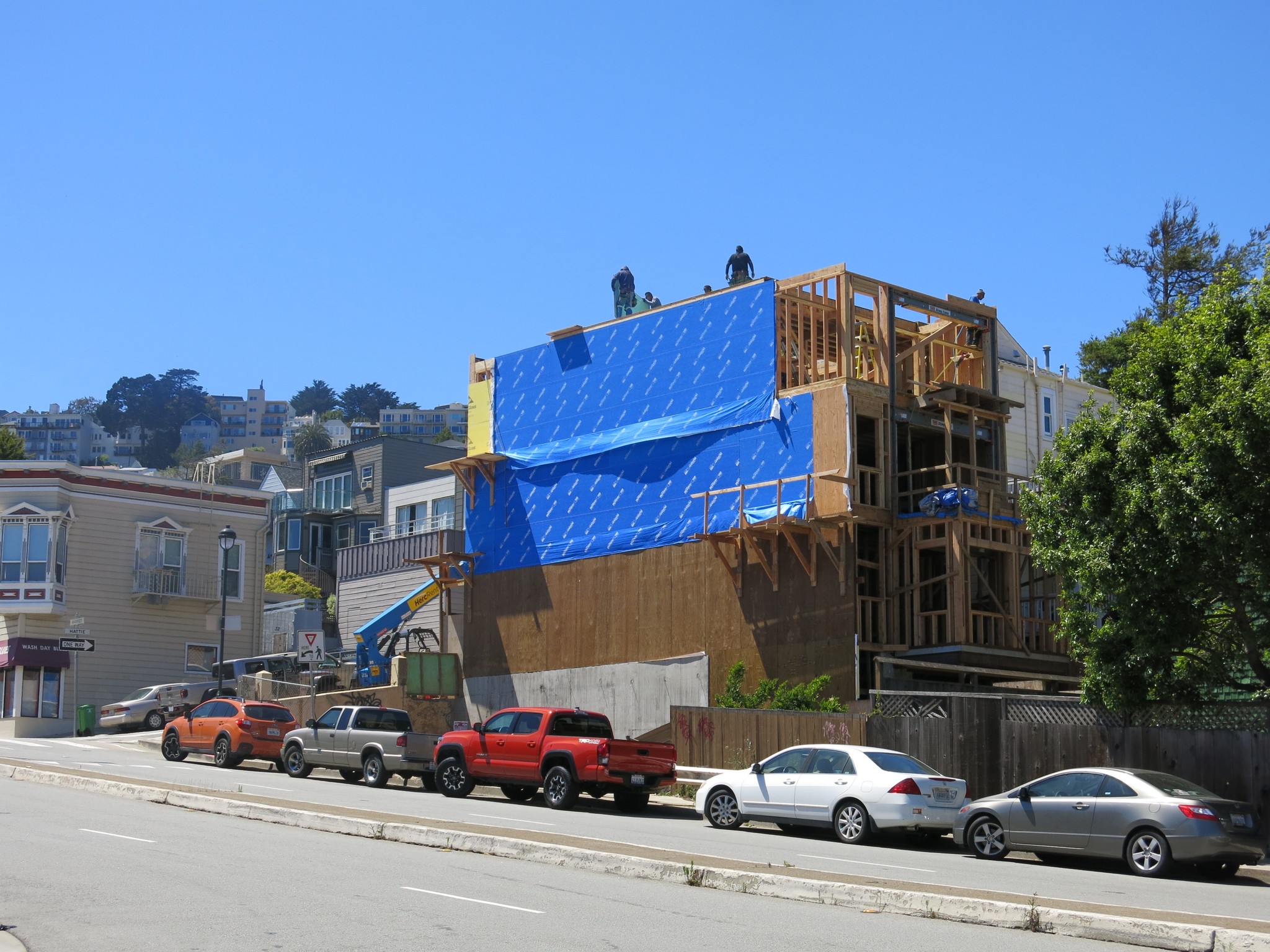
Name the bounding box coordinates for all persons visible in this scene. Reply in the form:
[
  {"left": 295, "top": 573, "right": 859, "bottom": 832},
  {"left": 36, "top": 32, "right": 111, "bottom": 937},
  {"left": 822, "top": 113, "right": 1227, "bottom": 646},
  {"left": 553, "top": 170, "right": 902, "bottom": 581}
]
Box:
[
  {"left": 611, "top": 266, "right": 635, "bottom": 318},
  {"left": 703, "top": 285, "right": 711, "bottom": 293},
  {"left": 964, "top": 289, "right": 985, "bottom": 354},
  {"left": 642, "top": 292, "right": 661, "bottom": 308},
  {"left": 726, "top": 245, "right": 755, "bottom": 285}
]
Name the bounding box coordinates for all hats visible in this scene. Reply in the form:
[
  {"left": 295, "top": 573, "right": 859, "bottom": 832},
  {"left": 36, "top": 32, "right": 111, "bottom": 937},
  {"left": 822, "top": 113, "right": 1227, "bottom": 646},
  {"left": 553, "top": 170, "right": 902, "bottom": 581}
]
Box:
[{"left": 704, "top": 285, "right": 711, "bottom": 289}]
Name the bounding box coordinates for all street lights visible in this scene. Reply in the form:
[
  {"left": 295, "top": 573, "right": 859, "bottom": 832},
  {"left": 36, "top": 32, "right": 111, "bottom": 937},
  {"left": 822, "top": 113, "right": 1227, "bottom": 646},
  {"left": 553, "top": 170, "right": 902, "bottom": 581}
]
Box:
[{"left": 216, "top": 524, "right": 237, "bottom": 697}]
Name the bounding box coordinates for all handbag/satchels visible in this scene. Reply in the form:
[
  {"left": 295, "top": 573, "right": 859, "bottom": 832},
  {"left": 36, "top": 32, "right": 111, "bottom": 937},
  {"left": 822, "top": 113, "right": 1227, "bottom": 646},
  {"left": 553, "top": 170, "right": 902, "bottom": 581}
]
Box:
[{"left": 632, "top": 295, "right": 638, "bottom": 307}]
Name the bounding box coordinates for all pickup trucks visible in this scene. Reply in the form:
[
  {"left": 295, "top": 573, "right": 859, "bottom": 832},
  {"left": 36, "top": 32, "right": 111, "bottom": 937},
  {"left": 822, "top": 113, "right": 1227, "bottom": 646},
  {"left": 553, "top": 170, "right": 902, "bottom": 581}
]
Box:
[
  {"left": 158, "top": 656, "right": 342, "bottom": 715},
  {"left": 433, "top": 708, "right": 678, "bottom": 811},
  {"left": 279, "top": 705, "right": 442, "bottom": 787}
]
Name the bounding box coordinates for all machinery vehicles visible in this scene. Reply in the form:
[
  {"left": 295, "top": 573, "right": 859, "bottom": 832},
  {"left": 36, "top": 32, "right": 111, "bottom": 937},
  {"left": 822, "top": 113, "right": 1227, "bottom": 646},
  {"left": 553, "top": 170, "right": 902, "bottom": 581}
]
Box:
[{"left": 354, "top": 562, "right": 470, "bottom": 689}]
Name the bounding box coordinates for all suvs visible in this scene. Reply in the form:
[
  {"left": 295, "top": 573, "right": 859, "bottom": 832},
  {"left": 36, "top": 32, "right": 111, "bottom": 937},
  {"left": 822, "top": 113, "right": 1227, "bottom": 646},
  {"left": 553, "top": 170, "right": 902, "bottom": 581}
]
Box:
[{"left": 161, "top": 696, "right": 302, "bottom": 772}]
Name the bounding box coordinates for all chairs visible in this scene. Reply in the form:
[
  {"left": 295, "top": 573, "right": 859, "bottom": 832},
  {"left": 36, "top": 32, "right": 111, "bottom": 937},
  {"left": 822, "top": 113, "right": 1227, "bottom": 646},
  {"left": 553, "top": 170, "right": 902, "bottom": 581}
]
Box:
[
  {"left": 382, "top": 718, "right": 396, "bottom": 730},
  {"left": 818, "top": 758, "right": 833, "bottom": 774},
  {"left": 554, "top": 719, "right": 567, "bottom": 733},
  {"left": 527, "top": 715, "right": 540, "bottom": 733}
]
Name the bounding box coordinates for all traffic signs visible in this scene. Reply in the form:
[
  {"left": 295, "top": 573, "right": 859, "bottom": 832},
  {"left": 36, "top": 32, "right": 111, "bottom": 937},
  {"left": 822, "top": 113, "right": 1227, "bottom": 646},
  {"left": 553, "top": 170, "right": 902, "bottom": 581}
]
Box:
[{"left": 59, "top": 637, "right": 95, "bottom": 653}]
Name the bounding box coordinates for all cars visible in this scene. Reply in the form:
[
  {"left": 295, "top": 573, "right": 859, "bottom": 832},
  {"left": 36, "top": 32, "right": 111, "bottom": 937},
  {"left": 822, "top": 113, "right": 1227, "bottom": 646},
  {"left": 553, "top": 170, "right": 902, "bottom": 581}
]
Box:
[
  {"left": 100, "top": 682, "right": 192, "bottom": 731},
  {"left": 263, "top": 652, "right": 357, "bottom": 691},
  {"left": 695, "top": 743, "right": 973, "bottom": 845},
  {"left": 953, "top": 766, "right": 1265, "bottom": 878}
]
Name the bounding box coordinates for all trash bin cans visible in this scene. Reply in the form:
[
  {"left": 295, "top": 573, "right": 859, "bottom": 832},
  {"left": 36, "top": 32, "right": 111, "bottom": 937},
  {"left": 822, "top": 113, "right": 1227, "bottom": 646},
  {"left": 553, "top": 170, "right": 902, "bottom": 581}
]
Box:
[{"left": 77, "top": 704, "right": 97, "bottom": 737}]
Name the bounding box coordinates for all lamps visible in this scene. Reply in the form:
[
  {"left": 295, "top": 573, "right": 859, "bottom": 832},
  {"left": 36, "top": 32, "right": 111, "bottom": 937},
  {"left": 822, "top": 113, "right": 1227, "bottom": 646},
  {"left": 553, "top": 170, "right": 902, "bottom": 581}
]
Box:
[{"left": 267, "top": 552, "right": 272, "bottom": 559}]
